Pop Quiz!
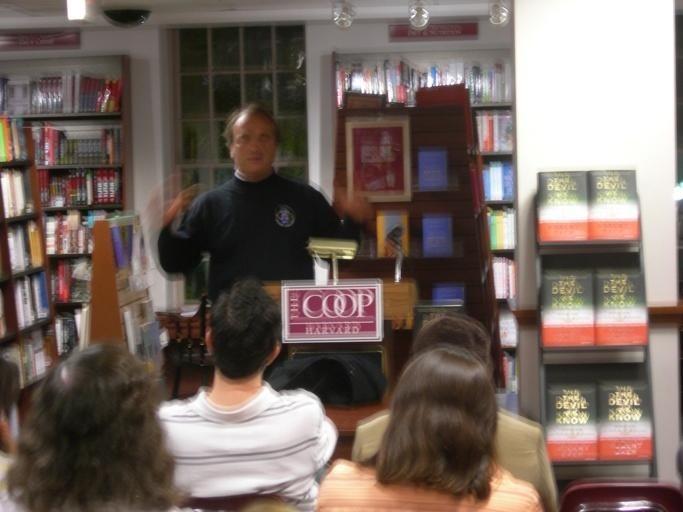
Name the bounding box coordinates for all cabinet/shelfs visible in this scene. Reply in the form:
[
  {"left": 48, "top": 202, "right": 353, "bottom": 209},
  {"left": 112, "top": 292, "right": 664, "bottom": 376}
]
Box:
[
  {"left": 1, "top": 53, "right": 137, "bottom": 459},
  {"left": 532, "top": 190, "right": 655, "bottom": 496},
  {"left": 329, "top": 48, "right": 515, "bottom": 390}
]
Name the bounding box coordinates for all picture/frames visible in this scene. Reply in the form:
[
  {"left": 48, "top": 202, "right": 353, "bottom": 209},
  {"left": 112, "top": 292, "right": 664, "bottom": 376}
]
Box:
[{"left": 288, "top": 341, "right": 391, "bottom": 407}]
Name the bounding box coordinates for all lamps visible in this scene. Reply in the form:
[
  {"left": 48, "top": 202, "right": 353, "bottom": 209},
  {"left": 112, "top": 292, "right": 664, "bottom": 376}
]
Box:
[{"left": 331, "top": 2, "right": 511, "bottom": 29}]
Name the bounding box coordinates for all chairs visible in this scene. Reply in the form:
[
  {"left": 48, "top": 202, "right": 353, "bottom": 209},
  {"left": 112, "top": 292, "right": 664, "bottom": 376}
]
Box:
[{"left": 561, "top": 482, "right": 681, "bottom": 510}]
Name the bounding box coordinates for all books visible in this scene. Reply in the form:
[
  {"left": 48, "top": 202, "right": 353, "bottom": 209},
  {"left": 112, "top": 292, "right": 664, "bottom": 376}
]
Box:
[
  {"left": 332, "top": 55, "right": 519, "bottom": 412},
  {"left": 0, "top": 71, "right": 168, "bottom": 393},
  {"left": 535, "top": 170, "right": 655, "bottom": 469}
]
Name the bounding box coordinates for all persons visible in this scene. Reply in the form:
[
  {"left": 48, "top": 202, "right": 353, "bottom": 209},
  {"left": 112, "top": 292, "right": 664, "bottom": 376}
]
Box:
[
  {"left": 155, "top": 104, "right": 369, "bottom": 379},
  {"left": 0, "top": 345, "right": 191, "bottom": 512},
  {"left": 316, "top": 344, "right": 543, "bottom": 512},
  {"left": 152, "top": 279, "right": 341, "bottom": 511},
  {"left": 349, "top": 314, "right": 561, "bottom": 511}
]
